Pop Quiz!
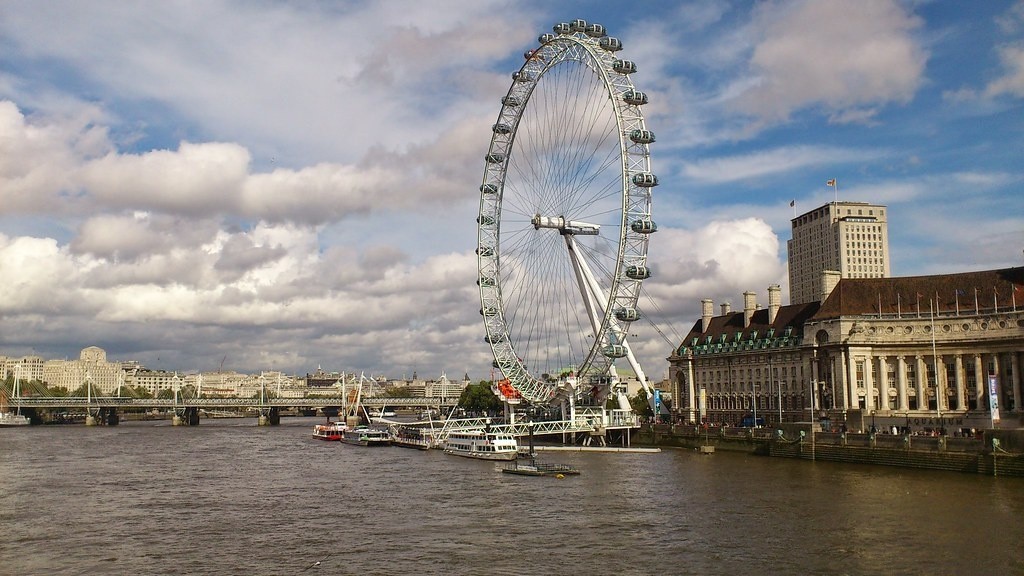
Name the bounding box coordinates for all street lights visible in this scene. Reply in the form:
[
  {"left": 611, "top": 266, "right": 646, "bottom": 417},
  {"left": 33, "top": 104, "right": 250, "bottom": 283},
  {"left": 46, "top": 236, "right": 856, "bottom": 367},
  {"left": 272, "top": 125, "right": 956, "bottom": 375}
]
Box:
[
  {"left": 872, "top": 409, "right": 876, "bottom": 432},
  {"left": 843, "top": 410, "right": 847, "bottom": 431},
  {"left": 809, "top": 380, "right": 818, "bottom": 422},
  {"left": 905, "top": 410, "right": 909, "bottom": 433},
  {"left": 752, "top": 385, "right": 760, "bottom": 426},
  {"left": 778, "top": 382, "right": 786, "bottom": 423}
]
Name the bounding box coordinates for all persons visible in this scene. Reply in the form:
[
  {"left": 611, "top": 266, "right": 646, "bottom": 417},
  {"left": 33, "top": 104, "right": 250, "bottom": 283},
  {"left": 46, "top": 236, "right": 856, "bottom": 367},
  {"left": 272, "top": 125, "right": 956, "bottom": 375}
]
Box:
[
  {"left": 871, "top": 425, "right": 978, "bottom": 436},
  {"left": 644, "top": 418, "right": 728, "bottom": 426},
  {"left": 343, "top": 429, "right": 345, "bottom": 435}
]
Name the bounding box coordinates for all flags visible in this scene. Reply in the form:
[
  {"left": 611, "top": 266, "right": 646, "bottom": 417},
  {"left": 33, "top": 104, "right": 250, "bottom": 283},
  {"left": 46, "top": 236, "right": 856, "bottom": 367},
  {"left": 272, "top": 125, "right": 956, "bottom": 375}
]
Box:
[
  {"left": 790, "top": 200, "right": 794, "bottom": 206},
  {"left": 994, "top": 289, "right": 1000, "bottom": 295},
  {"left": 1013, "top": 287, "right": 1019, "bottom": 294},
  {"left": 957, "top": 289, "right": 966, "bottom": 296},
  {"left": 827, "top": 177, "right": 836, "bottom": 187}
]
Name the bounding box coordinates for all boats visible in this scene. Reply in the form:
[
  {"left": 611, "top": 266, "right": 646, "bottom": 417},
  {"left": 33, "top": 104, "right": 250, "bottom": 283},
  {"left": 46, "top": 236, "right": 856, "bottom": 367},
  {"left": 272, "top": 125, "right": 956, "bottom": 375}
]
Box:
[
  {"left": 312, "top": 421, "right": 350, "bottom": 441},
  {"left": 340, "top": 426, "right": 391, "bottom": 446},
  {"left": 502, "top": 462, "right": 581, "bottom": 474},
  {"left": 391, "top": 426, "right": 434, "bottom": 451},
  {"left": 443, "top": 428, "right": 519, "bottom": 461}
]
